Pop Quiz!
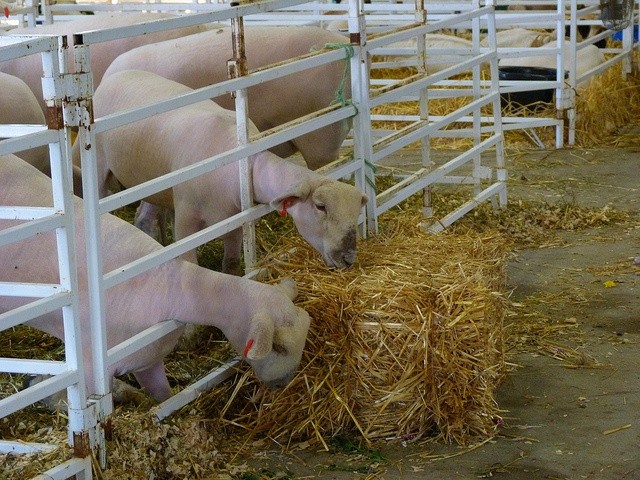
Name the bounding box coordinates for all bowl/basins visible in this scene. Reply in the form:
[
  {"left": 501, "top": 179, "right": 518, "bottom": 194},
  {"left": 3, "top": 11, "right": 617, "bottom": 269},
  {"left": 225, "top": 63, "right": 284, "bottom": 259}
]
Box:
[{"left": 491, "top": 66, "right": 568, "bottom": 117}]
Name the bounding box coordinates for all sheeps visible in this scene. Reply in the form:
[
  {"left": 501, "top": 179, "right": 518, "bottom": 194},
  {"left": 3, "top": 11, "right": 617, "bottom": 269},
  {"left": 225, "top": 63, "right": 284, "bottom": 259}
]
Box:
[
  {"left": 0, "top": 14, "right": 202, "bottom": 89},
  {"left": 498, "top": 35, "right": 605, "bottom": 80},
  {"left": 0, "top": 147, "right": 310, "bottom": 406},
  {"left": 479, "top": 25, "right": 552, "bottom": 50},
  {"left": 0, "top": 74, "right": 47, "bottom": 142},
  {"left": 90, "top": 68, "right": 368, "bottom": 274},
  {"left": 382, "top": 28, "right": 472, "bottom": 75},
  {"left": 70, "top": 28, "right": 373, "bottom": 233}
]
